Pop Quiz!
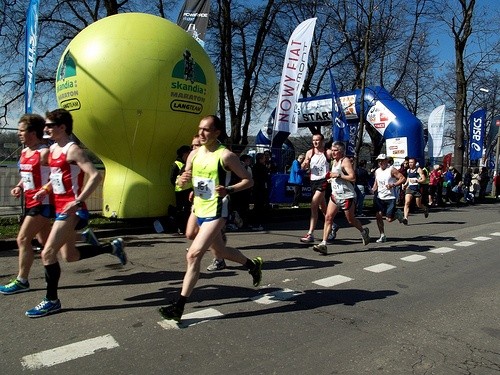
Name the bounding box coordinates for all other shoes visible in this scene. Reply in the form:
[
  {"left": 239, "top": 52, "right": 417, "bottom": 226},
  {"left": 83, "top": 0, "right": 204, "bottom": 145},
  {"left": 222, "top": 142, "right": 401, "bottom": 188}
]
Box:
[
  {"left": 293, "top": 206, "right": 300, "bottom": 208},
  {"left": 250, "top": 226, "right": 264, "bottom": 231}
]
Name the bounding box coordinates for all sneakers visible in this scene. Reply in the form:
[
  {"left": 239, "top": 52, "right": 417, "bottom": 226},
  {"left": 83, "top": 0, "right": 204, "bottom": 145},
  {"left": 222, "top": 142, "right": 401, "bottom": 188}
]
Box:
[
  {"left": 401, "top": 219, "right": 408, "bottom": 225},
  {"left": 328, "top": 224, "right": 339, "bottom": 239},
  {"left": 249, "top": 256, "right": 263, "bottom": 287},
  {"left": 361, "top": 227, "right": 369, "bottom": 246},
  {"left": 85, "top": 228, "right": 100, "bottom": 246},
  {"left": 158, "top": 305, "right": 183, "bottom": 322},
  {"left": 424, "top": 206, "right": 428, "bottom": 218},
  {"left": 113, "top": 237, "right": 129, "bottom": 265},
  {"left": 0, "top": 279, "right": 31, "bottom": 295},
  {"left": 376, "top": 236, "right": 387, "bottom": 243},
  {"left": 207, "top": 259, "right": 227, "bottom": 271},
  {"left": 396, "top": 209, "right": 402, "bottom": 222},
  {"left": 300, "top": 234, "right": 315, "bottom": 243},
  {"left": 25, "top": 298, "right": 61, "bottom": 318},
  {"left": 313, "top": 245, "right": 328, "bottom": 253}
]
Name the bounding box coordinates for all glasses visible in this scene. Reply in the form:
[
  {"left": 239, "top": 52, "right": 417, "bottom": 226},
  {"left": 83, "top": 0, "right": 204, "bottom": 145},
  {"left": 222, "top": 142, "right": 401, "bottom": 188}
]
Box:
[{"left": 44, "top": 122, "right": 56, "bottom": 128}]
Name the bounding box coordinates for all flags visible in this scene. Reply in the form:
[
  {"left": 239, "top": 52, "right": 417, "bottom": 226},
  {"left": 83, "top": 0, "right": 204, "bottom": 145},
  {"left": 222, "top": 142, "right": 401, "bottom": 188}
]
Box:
[
  {"left": 442, "top": 153, "right": 452, "bottom": 171},
  {"left": 177, "top": 0, "right": 210, "bottom": 48},
  {"left": 425, "top": 104, "right": 446, "bottom": 157},
  {"left": 327, "top": 63, "right": 351, "bottom": 142},
  {"left": 23, "top": 0, "right": 40, "bottom": 108},
  {"left": 275, "top": 17, "right": 317, "bottom": 130},
  {"left": 470, "top": 111, "right": 486, "bottom": 161}
]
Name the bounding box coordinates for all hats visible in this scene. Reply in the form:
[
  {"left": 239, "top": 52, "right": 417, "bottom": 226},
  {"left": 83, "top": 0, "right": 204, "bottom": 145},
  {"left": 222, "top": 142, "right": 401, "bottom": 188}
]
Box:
[{"left": 376, "top": 154, "right": 387, "bottom": 160}]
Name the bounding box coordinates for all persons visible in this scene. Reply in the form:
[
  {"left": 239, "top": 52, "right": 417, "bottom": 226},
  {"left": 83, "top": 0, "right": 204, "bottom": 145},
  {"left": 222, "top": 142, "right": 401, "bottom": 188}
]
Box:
[
  {"left": 372, "top": 154, "right": 404, "bottom": 242},
  {"left": 354, "top": 158, "right": 368, "bottom": 216},
  {"left": 186, "top": 134, "right": 228, "bottom": 270},
  {"left": 402, "top": 158, "right": 428, "bottom": 225},
  {"left": 301, "top": 134, "right": 340, "bottom": 241},
  {"left": 231, "top": 151, "right": 270, "bottom": 230},
  {"left": 313, "top": 140, "right": 369, "bottom": 256},
  {"left": 158, "top": 116, "right": 261, "bottom": 322},
  {"left": 26, "top": 110, "right": 127, "bottom": 318},
  {"left": 428, "top": 165, "right": 500, "bottom": 206},
  {"left": 0, "top": 114, "right": 97, "bottom": 295},
  {"left": 172, "top": 146, "right": 191, "bottom": 234},
  {"left": 288, "top": 154, "right": 310, "bottom": 209}
]
